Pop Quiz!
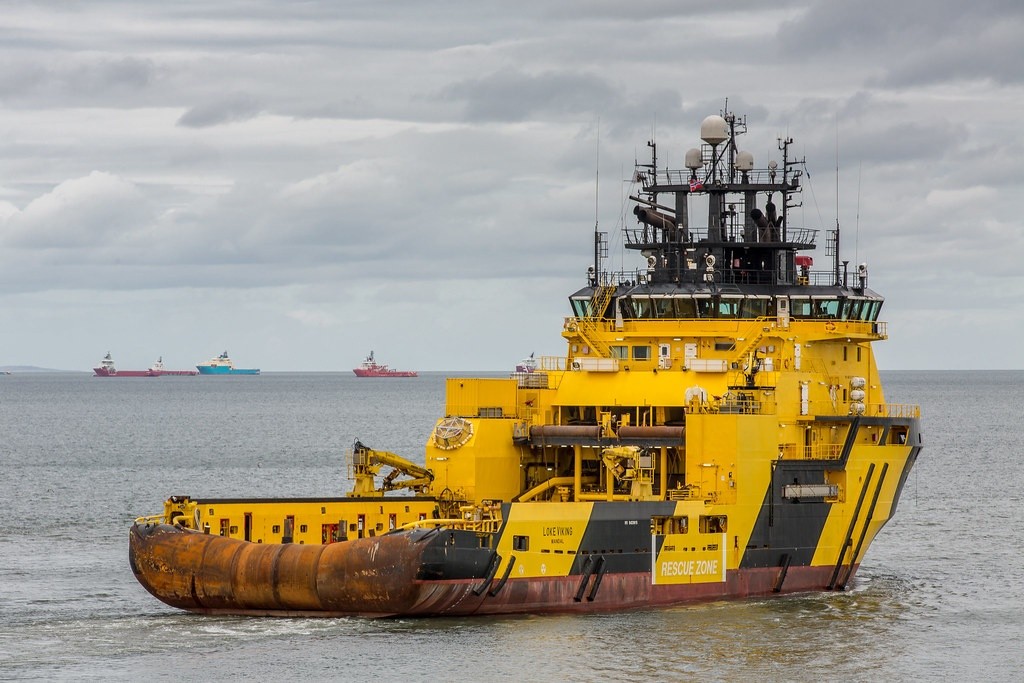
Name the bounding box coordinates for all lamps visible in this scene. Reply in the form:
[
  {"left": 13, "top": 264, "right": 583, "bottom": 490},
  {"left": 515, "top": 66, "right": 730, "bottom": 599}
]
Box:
[
  {"left": 859, "top": 263, "right": 867, "bottom": 277},
  {"left": 647, "top": 255, "right": 657, "bottom": 273},
  {"left": 587, "top": 265, "right": 595, "bottom": 275},
  {"left": 705, "top": 255, "right": 715, "bottom": 272}
]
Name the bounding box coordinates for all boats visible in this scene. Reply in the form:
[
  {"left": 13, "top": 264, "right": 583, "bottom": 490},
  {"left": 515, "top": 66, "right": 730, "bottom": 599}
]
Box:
[
  {"left": 93, "top": 354, "right": 200, "bottom": 377},
  {"left": 128, "top": 97, "right": 924, "bottom": 618},
  {"left": 196, "top": 352, "right": 260, "bottom": 375},
  {"left": 353, "top": 351, "right": 418, "bottom": 377}
]
